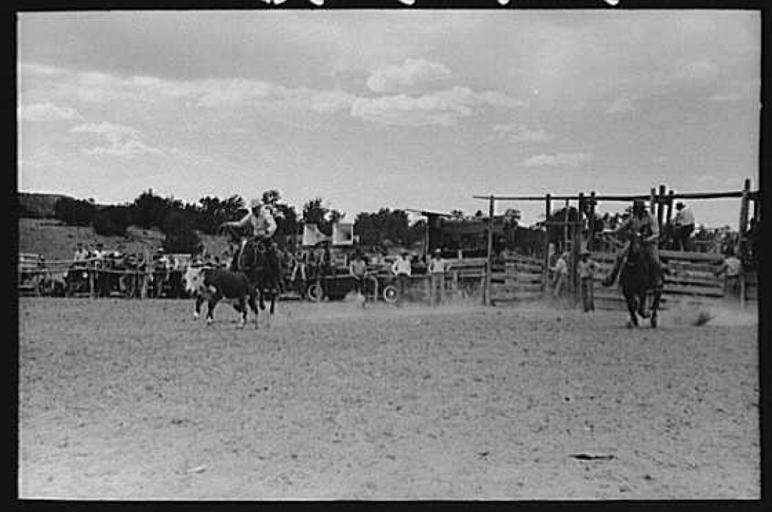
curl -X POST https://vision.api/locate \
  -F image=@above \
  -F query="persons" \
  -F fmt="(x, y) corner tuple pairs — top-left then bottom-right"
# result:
(553, 239), (602, 320)
(426, 246), (453, 308)
(710, 218), (757, 301)
(223, 197), (277, 241)
(18, 244), (238, 299)
(671, 202), (695, 255)
(604, 198), (678, 285)
(280, 239), (423, 303)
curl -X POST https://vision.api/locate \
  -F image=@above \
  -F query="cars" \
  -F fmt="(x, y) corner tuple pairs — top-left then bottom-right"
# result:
(304, 263), (399, 304)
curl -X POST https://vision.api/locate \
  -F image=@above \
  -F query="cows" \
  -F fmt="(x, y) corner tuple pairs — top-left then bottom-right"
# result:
(181, 262), (260, 331)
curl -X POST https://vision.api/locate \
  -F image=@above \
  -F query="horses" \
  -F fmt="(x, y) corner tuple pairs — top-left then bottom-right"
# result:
(619, 223), (663, 330)
(228, 234), (279, 317)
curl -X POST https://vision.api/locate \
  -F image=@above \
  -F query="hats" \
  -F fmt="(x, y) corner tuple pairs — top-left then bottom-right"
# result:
(249, 198), (263, 207)
(629, 200), (649, 207)
